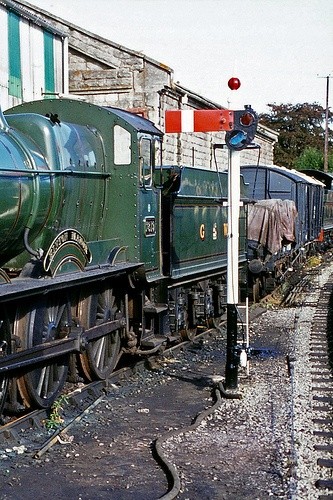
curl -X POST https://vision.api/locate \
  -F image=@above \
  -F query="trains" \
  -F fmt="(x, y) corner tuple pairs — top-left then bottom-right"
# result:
(0, 96), (333, 407)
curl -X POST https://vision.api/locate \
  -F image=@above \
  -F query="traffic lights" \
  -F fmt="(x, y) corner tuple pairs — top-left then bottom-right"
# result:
(224, 110), (256, 148)
